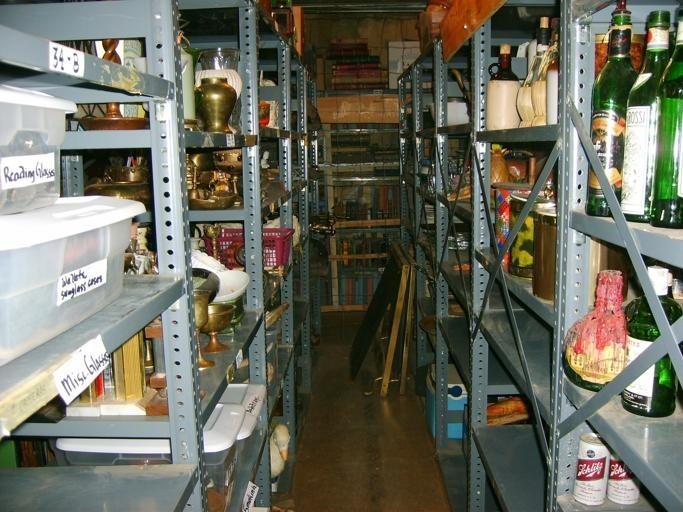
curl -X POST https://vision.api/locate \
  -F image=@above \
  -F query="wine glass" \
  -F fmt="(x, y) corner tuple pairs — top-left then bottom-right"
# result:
(193, 289), (234, 370)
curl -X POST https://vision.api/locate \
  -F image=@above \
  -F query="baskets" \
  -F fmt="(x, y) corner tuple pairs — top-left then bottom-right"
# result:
(200, 228), (295, 270)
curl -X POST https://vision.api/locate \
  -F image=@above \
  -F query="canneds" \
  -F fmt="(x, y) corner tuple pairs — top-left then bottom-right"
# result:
(607, 450), (640, 506)
(573, 433), (612, 507)
(492, 180), (558, 304)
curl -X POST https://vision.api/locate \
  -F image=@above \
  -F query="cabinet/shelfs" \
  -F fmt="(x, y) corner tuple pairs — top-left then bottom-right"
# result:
(0, 4), (322, 511)
(316, 99), (404, 314)
(393, 2), (683, 512)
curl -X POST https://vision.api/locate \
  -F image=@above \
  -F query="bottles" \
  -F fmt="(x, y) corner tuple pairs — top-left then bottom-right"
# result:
(582, 1), (683, 230)
(487, 16), (561, 128)
(560, 255), (683, 418)
(195, 78), (237, 132)
